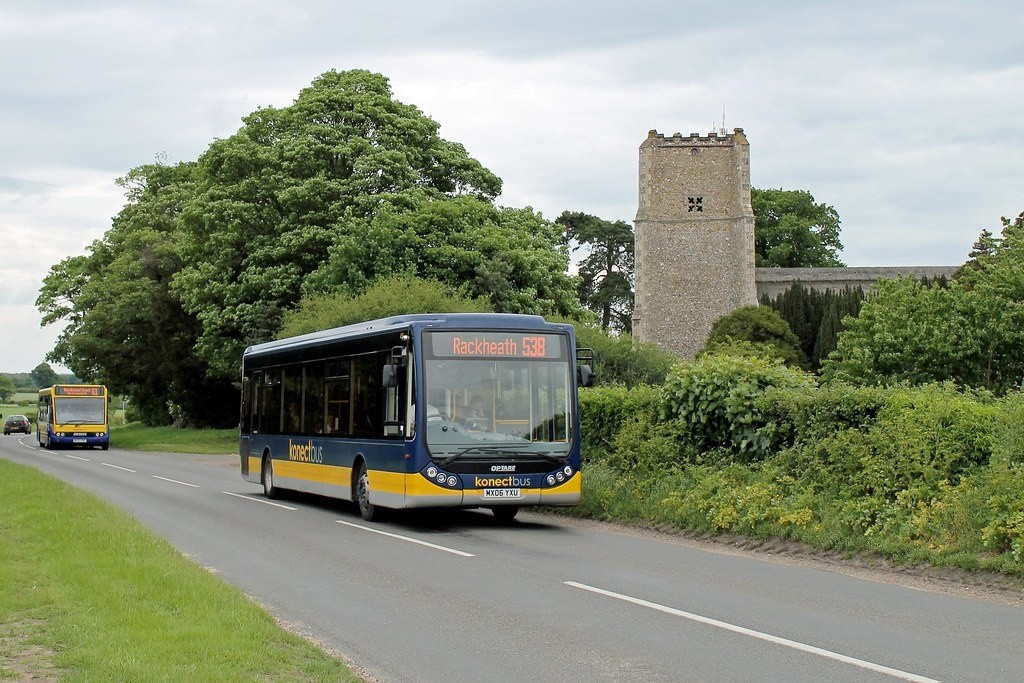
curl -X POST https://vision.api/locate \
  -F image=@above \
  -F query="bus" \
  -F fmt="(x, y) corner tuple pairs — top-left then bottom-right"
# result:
(35, 383), (112, 451)
(237, 311), (596, 527)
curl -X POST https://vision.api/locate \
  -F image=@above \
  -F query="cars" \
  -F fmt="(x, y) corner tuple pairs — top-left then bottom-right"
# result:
(3, 414), (33, 436)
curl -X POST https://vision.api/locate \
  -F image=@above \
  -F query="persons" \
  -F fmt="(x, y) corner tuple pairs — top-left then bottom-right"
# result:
(409, 402), (440, 430)
(286, 375), (336, 434)
(461, 394), (488, 431)
